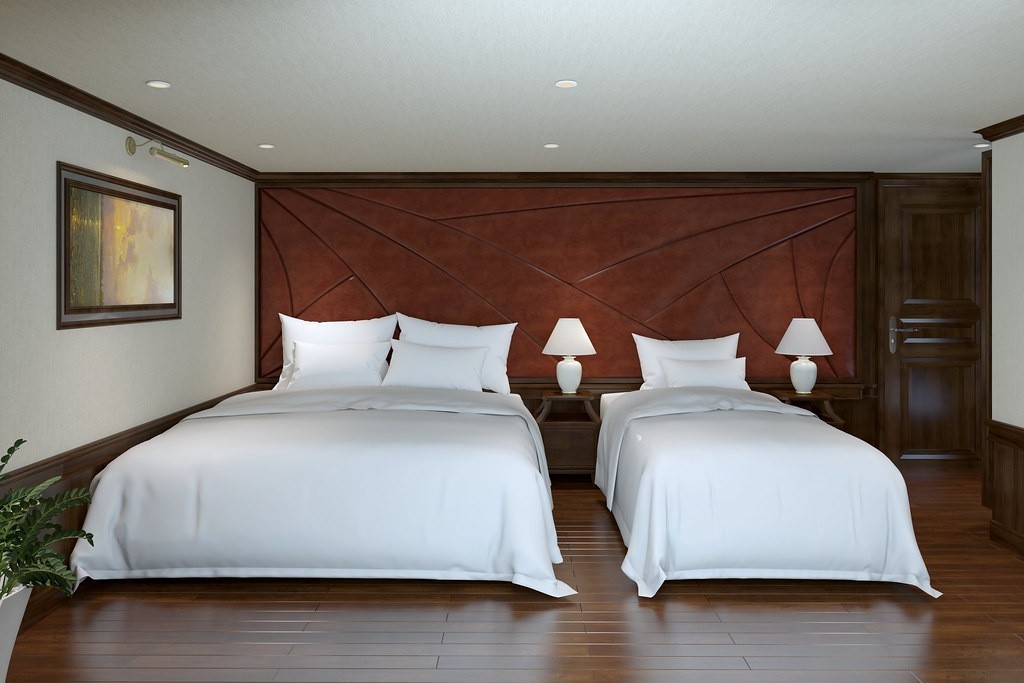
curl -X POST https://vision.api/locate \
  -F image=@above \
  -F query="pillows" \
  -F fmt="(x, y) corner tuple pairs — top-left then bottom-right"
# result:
(286, 341), (392, 389)
(381, 338), (490, 392)
(273, 312), (397, 395)
(658, 356), (753, 391)
(396, 311), (521, 395)
(629, 331), (741, 391)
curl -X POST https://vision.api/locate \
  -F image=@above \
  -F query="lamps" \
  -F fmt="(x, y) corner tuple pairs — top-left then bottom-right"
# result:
(125, 134), (190, 168)
(774, 318), (833, 393)
(542, 318), (597, 394)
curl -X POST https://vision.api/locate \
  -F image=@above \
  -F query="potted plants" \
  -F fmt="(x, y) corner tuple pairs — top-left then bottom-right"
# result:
(0, 439), (94, 683)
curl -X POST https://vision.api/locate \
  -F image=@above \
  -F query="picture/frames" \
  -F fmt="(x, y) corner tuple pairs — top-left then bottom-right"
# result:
(55, 160), (183, 328)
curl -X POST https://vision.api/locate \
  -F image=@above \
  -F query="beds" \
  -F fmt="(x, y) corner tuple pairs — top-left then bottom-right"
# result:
(68, 389), (578, 601)
(593, 387), (943, 601)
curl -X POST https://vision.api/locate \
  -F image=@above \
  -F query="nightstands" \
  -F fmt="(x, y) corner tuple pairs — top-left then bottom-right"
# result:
(774, 388), (847, 430)
(532, 390), (603, 487)
